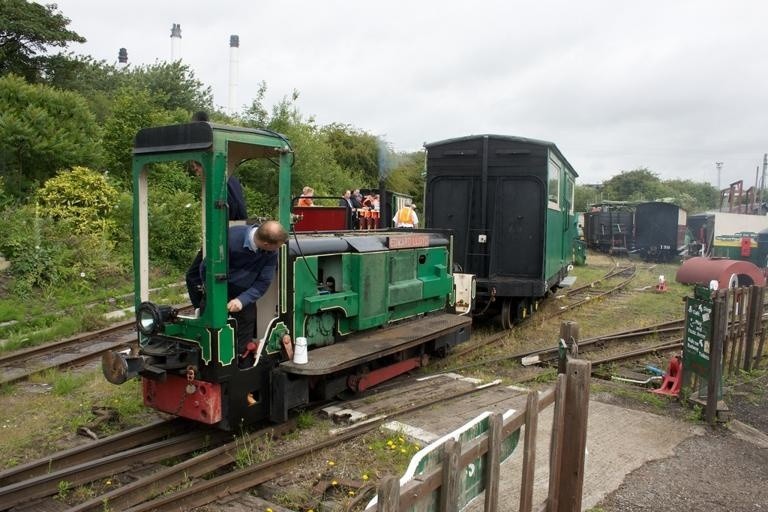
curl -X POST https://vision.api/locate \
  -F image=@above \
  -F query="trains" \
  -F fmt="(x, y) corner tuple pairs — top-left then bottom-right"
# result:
(582, 202), (688, 260)
(104, 120), (587, 429)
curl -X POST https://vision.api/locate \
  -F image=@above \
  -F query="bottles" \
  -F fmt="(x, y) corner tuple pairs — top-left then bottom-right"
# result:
(291, 337), (310, 366)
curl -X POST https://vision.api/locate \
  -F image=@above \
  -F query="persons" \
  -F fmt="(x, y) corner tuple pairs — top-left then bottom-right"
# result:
(390, 203), (419, 229)
(338, 188), (381, 230)
(296, 185), (324, 208)
(325, 276), (336, 293)
(186, 218), (290, 407)
